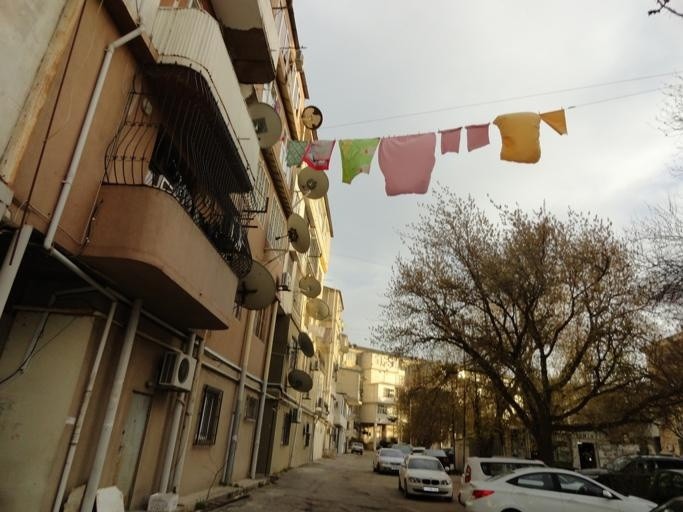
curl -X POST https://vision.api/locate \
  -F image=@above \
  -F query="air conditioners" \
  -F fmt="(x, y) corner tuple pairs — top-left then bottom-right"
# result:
(156, 350), (198, 392)
(289, 407), (302, 423)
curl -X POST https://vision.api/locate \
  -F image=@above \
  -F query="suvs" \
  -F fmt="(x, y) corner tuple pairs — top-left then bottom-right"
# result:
(574, 453), (683, 501)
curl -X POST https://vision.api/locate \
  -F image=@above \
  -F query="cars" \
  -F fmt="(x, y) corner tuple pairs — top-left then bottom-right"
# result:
(351, 442), (364, 455)
(398, 453), (454, 501)
(464, 465), (659, 512)
(372, 447), (405, 474)
